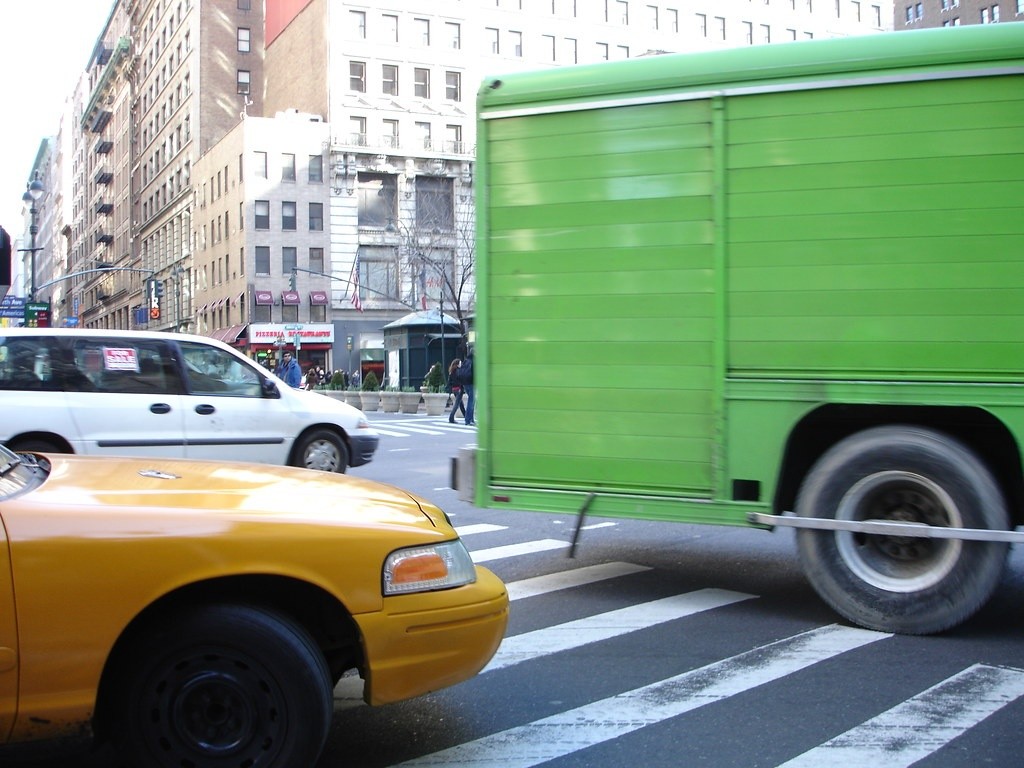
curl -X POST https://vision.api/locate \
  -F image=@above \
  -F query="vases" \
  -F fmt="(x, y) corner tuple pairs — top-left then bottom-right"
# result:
(450, 394), (468, 417)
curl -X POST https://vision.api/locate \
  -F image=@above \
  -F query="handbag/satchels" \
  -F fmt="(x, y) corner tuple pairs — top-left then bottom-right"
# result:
(447, 396), (453, 407)
(456, 360), (473, 381)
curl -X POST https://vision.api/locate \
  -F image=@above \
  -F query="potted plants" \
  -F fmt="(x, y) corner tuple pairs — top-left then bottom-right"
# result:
(419, 381), (429, 393)
(310, 385), (327, 396)
(397, 386), (422, 414)
(379, 386), (402, 412)
(325, 372), (345, 402)
(421, 361), (450, 416)
(344, 383), (362, 410)
(359, 370), (383, 411)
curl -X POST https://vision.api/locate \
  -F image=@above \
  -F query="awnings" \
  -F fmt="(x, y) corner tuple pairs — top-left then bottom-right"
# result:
(211, 324), (247, 342)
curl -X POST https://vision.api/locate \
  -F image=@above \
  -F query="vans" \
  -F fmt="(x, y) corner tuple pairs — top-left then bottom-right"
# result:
(0, 323), (381, 476)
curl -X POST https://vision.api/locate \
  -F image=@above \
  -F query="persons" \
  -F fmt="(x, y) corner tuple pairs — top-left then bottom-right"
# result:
(461, 348), (476, 426)
(448, 359), (466, 423)
(279, 350), (302, 388)
(306, 365), (360, 390)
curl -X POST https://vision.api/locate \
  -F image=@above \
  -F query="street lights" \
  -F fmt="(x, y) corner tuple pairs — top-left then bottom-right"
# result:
(21, 169), (44, 304)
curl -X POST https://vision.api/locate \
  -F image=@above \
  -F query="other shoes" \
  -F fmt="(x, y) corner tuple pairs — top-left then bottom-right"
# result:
(449, 421), (458, 424)
(465, 422), (474, 426)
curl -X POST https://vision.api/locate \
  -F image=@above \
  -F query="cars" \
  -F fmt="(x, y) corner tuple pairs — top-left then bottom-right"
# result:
(0, 436), (513, 766)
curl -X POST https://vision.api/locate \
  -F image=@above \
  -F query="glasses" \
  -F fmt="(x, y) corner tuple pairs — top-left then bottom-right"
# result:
(284, 354), (291, 357)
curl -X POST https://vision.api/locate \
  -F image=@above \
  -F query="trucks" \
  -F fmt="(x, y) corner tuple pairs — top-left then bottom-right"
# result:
(444, 19), (1024, 636)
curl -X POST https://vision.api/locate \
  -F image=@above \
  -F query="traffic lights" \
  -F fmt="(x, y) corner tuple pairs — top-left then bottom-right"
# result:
(289, 274), (296, 291)
(155, 279), (163, 298)
